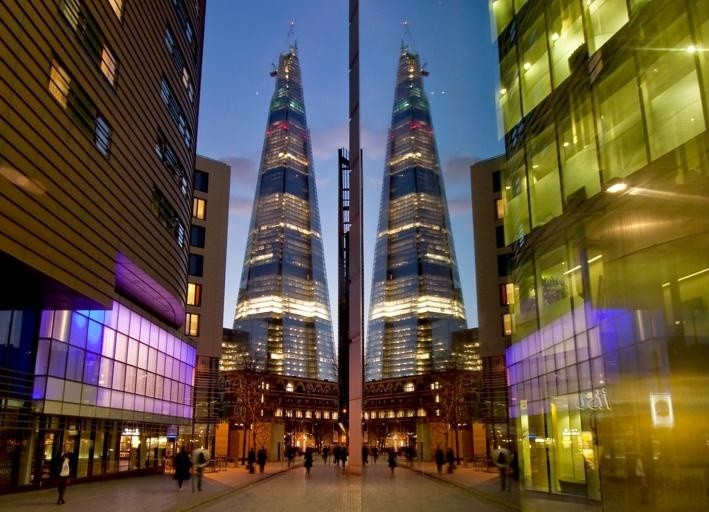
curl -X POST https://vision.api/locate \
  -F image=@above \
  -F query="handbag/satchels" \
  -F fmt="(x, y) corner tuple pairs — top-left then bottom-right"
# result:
(199, 449), (207, 464)
(247, 465), (249, 469)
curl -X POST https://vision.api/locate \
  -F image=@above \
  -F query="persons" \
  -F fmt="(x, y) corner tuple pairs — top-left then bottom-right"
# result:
(247, 448), (256, 474)
(434, 446), (445, 474)
(256, 446), (267, 474)
(53, 443), (75, 505)
(493, 446), (512, 492)
(284, 445), (417, 478)
(509, 448), (518, 484)
(172, 445), (193, 491)
(446, 448), (455, 474)
(191, 442), (210, 493)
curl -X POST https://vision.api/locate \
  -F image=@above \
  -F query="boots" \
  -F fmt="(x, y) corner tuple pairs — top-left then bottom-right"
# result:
(58, 490), (65, 504)
